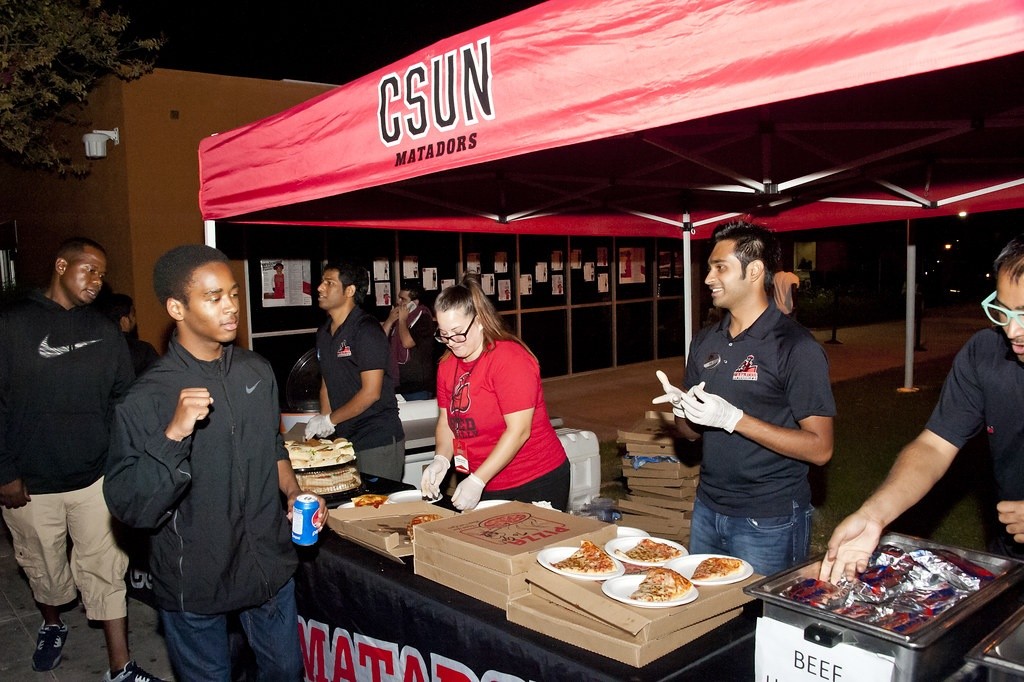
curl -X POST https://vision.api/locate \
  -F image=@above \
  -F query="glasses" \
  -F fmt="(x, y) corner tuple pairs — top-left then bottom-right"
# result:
(433, 313), (477, 343)
(981, 290), (1024, 329)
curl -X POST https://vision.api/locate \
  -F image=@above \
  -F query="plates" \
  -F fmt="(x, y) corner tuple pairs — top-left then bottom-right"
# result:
(605, 537), (689, 570)
(661, 553), (755, 585)
(601, 575), (702, 608)
(337, 500), (393, 509)
(536, 545), (626, 581)
(387, 490), (443, 504)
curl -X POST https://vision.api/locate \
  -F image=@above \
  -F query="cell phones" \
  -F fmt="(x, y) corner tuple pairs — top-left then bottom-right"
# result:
(407, 301), (416, 313)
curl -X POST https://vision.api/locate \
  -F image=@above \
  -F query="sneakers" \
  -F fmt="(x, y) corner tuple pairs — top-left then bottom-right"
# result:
(31, 619), (70, 671)
(104, 658), (168, 682)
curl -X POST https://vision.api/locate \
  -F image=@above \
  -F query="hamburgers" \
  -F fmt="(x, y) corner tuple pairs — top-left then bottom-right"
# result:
(282, 438), (355, 469)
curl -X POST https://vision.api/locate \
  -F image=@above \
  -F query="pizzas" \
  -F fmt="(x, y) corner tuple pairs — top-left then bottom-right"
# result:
(691, 558), (742, 580)
(615, 538), (682, 562)
(550, 540), (617, 573)
(632, 567), (692, 602)
(352, 494), (388, 508)
(407, 514), (442, 542)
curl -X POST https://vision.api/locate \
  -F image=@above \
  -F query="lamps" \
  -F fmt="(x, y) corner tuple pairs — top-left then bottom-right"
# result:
(81, 126), (120, 159)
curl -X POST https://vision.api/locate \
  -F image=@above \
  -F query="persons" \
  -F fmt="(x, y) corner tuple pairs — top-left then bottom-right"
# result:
(771, 256), (800, 315)
(103, 244), (329, 682)
(383, 281), (440, 401)
(420, 275), (570, 512)
(313, 259), (406, 481)
(0, 237), (162, 682)
(819, 236), (1024, 586)
(652, 220), (835, 576)
(104, 293), (160, 370)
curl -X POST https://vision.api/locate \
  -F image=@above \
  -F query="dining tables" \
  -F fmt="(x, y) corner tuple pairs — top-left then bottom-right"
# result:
(111, 472), (419, 610)
(661, 629), (756, 682)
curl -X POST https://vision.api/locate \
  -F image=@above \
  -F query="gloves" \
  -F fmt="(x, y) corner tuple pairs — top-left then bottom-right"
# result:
(314, 427), (336, 439)
(678, 386), (747, 435)
(450, 473), (487, 512)
(649, 370), (708, 419)
(421, 455), (453, 500)
(304, 413), (338, 440)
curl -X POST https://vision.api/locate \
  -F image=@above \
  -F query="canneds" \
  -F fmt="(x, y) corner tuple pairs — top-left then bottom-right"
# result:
(292, 494), (319, 546)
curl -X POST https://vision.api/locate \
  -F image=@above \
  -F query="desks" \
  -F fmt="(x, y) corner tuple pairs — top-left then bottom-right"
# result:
(296, 470), (757, 682)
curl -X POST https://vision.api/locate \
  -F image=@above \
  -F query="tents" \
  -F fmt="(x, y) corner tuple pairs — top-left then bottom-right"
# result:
(198, 0), (1024, 386)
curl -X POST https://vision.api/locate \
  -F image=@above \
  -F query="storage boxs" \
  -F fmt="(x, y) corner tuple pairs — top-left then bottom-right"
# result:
(325, 500), (462, 564)
(527, 563), (765, 639)
(414, 559), (527, 610)
(554, 427), (601, 514)
(615, 410), (700, 549)
(505, 593), (744, 668)
(743, 530), (1024, 682)
(963, 606), (1024, 682)
(413, 543), (528, 594)
(414, 500), (617, 576)
